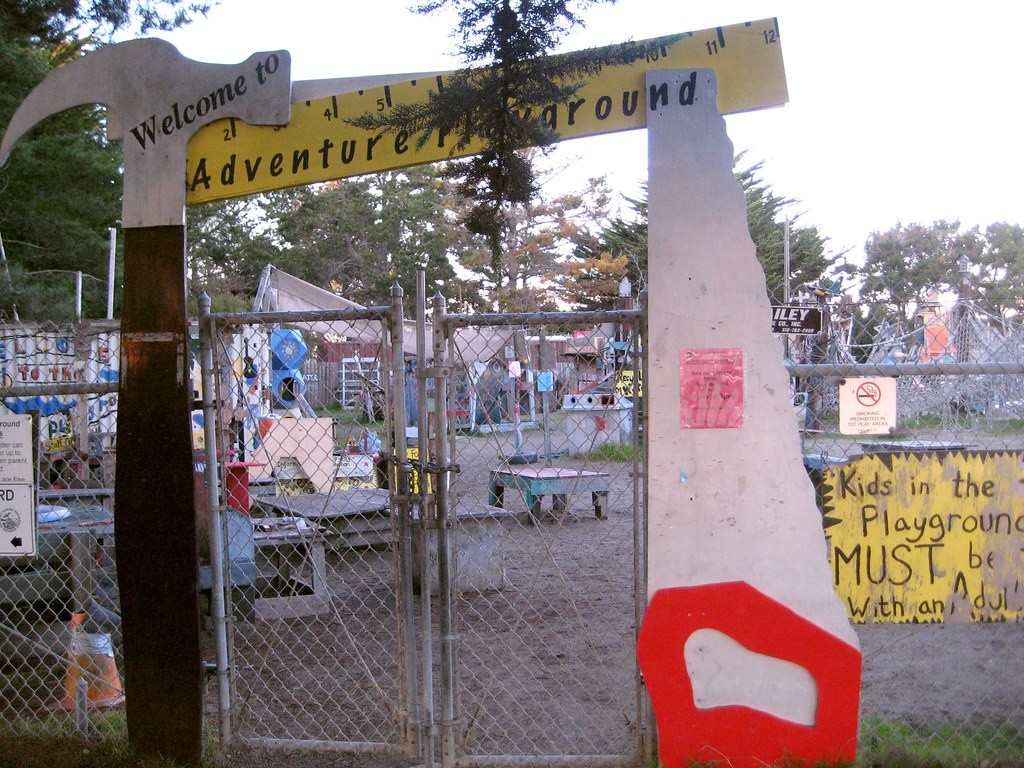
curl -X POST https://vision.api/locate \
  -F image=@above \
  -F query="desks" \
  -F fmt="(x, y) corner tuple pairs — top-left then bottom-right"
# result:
(249, 517), (334, 621)
(0, 506), (115, 689)
(859, 440), (977, 455)
(490, 469), (610, 525)
(384, 503), (525, 592)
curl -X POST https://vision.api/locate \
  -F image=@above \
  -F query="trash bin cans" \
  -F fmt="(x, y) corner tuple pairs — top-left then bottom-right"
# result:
(792, 391), (824, 435)
(562, 393), (634, 460)
(858, 439), (978, 452)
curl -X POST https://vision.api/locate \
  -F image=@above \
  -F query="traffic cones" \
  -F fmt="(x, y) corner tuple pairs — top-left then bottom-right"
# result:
(54, 592), (124, 709)
